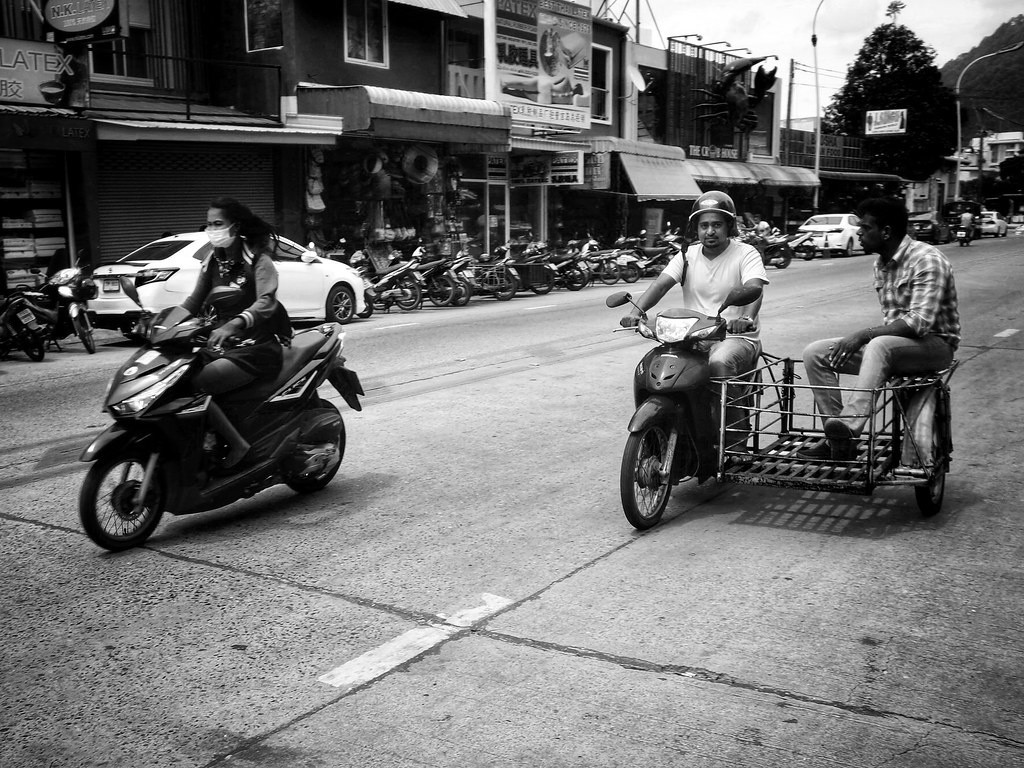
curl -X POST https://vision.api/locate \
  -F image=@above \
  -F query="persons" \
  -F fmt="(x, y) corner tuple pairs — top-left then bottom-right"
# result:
(179, 195), (293, 475)
(736, 214), (782, 240)
(961, 207), (975, 237)
(795, 196), (962, 458)
(868, 112), (872, 130)
(620, 190), (769, 463)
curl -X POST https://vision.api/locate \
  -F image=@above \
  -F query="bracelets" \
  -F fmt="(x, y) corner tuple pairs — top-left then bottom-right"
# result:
(739, 316), (753, 321)
(866, 328), (868, 337)
(869, 327), (873, 337)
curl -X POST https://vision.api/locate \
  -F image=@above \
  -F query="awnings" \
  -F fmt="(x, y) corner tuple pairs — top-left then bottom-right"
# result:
(595, 151), (705, 202)
(685, 156), (759, 185)
(811, 168), (902, 182)
(0, 103), (343, 145)
(392, 0), (471, 17)
(740, 161), (822, 186)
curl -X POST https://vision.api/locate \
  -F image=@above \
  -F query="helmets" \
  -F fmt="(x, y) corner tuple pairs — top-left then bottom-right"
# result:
(688, 190), (738, 237)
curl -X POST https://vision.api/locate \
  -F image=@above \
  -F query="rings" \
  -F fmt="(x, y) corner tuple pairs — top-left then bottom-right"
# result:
(841, 353), (846, 356)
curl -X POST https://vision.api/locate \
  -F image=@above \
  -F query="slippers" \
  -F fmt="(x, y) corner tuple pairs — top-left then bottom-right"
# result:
(727, 445), (752, 464)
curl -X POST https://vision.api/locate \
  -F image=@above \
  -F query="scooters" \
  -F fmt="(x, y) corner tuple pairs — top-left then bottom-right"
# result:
(954, 218), (978, 246)
(306, 218), (817, 319)
(0, 248), (95, 364)
(78, 274), (367, 550)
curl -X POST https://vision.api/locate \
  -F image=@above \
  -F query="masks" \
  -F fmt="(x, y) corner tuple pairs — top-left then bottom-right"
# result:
(205, 223), (236, 248)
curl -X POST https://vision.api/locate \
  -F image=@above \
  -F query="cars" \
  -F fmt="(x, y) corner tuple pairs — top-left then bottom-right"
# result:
(905, 210), (956, 245)
(793, 213), (873, 257)
(84, 230), (368, 346)
(942, 206), (1010, 247)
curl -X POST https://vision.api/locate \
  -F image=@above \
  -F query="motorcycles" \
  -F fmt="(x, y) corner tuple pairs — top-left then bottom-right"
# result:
(607, 190), (963, 531)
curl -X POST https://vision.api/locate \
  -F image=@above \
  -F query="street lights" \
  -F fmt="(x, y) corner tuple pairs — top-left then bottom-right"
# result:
(953, 40), (1024, 203)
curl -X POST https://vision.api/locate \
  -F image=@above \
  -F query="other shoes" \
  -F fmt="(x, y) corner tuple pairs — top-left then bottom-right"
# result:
(823, 417), (856, 462)
(797, 441), (830, 459)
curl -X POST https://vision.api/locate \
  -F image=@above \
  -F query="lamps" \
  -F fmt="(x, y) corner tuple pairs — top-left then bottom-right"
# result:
(764, 55), (778, 60)
(720, 48), (751, 53)
(700, 41), (731, 47)
(668, 34), (702, 40)
